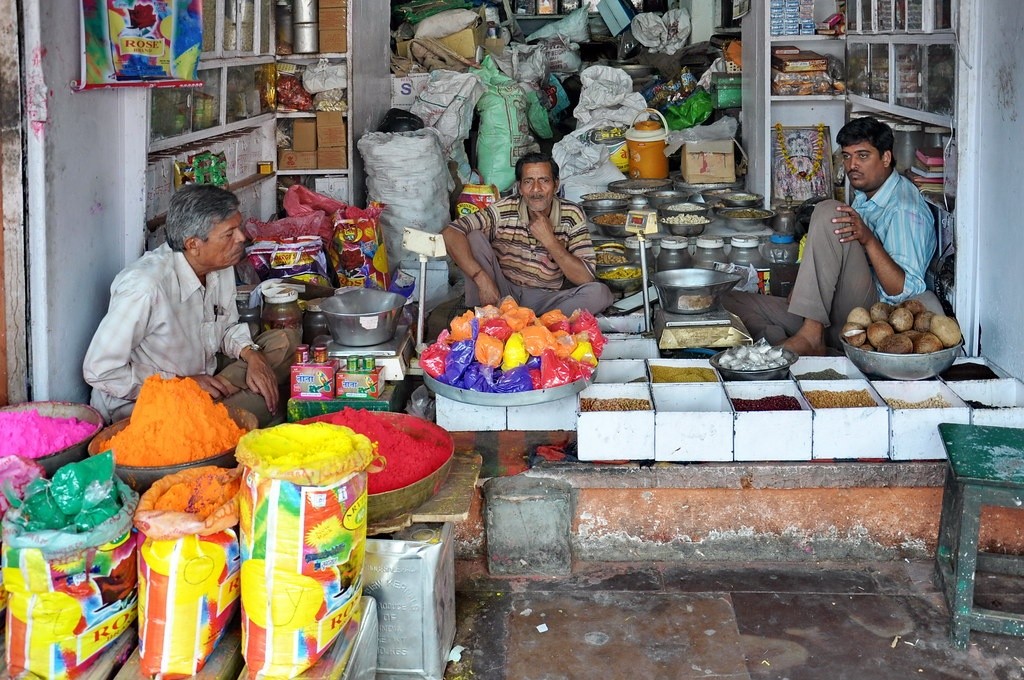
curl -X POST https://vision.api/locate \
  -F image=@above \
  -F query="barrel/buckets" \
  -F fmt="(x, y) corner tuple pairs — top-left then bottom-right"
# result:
(625, 108), (670, 179)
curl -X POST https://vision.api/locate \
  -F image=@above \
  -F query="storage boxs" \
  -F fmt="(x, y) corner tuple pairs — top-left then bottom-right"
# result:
(147, 0), (1024, 462)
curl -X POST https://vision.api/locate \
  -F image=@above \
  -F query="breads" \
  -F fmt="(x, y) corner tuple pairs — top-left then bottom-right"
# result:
(842, 299), (962, 355)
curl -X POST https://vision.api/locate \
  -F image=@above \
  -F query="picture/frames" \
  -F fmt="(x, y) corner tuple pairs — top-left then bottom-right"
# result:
(771, 126), (836, 207)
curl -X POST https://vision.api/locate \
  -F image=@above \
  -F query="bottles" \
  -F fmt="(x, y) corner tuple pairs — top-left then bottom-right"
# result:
(625, 204), (807, 296)
(275, 0), (292, 55)
(235, 288), (330, 348)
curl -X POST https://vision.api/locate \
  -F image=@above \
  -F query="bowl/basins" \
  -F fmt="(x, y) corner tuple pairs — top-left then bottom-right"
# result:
(577, 170), (777, 237)
(0, 401), (104, 479)
(614, 65), (654, 78)
(648, 269), (745, 315)
(288, 409), (454, 525)
(710, 347), (799, 382)
(319, 289), (407, 347)
(593, 242), (648, 293)
(88, 407), (259, 496)
(838, 333), (963, 381)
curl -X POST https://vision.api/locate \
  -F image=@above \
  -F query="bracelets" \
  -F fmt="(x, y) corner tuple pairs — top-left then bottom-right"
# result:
(472, 268), (483, 281)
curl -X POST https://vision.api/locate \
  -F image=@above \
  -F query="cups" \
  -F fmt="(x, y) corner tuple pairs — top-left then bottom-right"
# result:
(293, 23), (319, 54)
(292, 0), (320, 23)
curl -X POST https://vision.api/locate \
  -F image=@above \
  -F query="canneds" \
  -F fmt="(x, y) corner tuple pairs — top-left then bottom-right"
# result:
(346, 355), (375, 371)
(296, 344), (327, 363)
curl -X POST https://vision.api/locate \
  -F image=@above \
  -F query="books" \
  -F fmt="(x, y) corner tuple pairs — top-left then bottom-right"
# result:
(904, 147), (943, 194)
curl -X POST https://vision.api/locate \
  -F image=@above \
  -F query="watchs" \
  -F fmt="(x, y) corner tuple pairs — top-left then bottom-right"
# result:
(242, 344), (263, 362)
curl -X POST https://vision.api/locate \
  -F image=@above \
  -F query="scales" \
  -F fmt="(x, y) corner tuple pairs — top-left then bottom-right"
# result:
(624, 208), (753, 355)
(327, 226), (449, 381)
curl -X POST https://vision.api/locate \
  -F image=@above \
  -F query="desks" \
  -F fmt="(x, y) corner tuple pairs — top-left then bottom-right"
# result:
(935, 422), (1024, 649)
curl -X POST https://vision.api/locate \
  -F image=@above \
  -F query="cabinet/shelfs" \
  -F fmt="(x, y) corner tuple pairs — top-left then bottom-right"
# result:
(125, 0), (277, 266)
(742, 0), (845, 200)
(845, 0), (963, 130)
(277, 0), (392, 208)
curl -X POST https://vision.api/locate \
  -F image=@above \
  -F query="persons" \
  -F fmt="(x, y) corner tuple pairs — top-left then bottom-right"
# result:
(439, 151), (614, 318)
(83, 184), (300, 428)
(722, 117), (937, 357)
